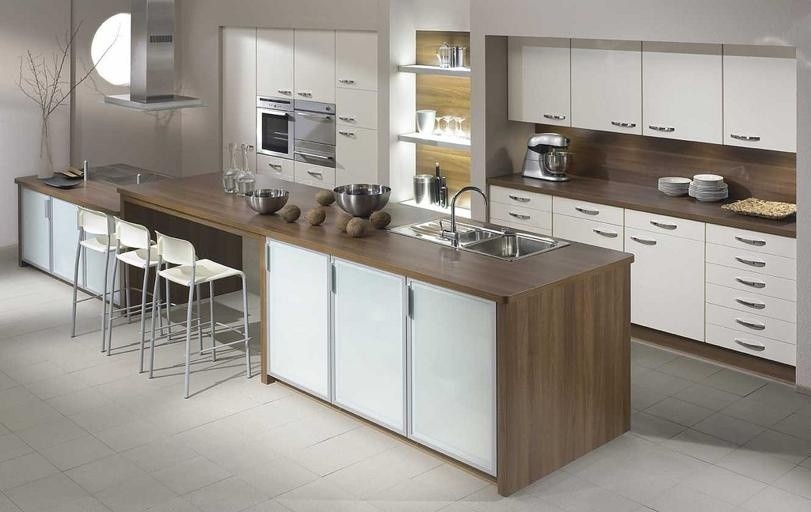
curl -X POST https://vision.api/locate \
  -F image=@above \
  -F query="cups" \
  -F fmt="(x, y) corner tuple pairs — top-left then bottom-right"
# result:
(416, 109), (436, 136)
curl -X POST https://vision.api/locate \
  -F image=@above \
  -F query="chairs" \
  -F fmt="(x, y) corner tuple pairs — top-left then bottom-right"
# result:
(69, 203), (255, 400)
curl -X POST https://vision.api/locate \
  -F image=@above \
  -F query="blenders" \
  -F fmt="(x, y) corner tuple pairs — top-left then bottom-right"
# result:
(520, 133), (573, 183)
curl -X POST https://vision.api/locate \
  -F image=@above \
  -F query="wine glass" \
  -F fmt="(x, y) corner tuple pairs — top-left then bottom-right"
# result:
(434, 115), (467, 138)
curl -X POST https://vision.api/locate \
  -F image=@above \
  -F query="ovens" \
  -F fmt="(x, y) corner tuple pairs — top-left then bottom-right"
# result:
(256, 95), (336, 170)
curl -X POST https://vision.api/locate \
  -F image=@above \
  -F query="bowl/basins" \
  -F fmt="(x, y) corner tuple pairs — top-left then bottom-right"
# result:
(333, 183), (392, 217)
(242, 189), (290, 214)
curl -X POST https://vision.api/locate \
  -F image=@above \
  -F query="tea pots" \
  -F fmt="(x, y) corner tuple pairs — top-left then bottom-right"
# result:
(433, 42), (452, 69)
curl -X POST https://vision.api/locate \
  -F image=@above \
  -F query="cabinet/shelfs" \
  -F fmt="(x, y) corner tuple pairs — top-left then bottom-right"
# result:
(15, 184), (53, 274)
(78, 202), (122, 308)
(257, 153), (337, 192)
(550, 198), (705, 348)
(328, 258), (409, 436)
(263, 238), (332, 410)
(256, 31), (335, 104)
(724, 44), (798, 148)
(51, 192), (85, 290)
(704, 218), (798, 371)
(507, 39), (572, 126)
(396, 58), (471, 221)
(336, 29), (380, 92)
(337, 87), (378, 188)
(486, 184), (550, 241)
(404, 261), (634, 475)
(571, 44), (723, 144)
(219, 27), (256, 180)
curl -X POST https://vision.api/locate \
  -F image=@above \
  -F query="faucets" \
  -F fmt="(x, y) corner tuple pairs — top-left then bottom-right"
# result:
(438, 186), (487, 246)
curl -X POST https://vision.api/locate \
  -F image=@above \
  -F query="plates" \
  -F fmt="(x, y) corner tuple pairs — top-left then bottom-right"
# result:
(657, 174), (729, 203)
(43, 177), (82, 188)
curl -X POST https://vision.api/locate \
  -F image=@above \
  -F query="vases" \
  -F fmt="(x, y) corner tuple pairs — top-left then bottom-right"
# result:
(33, 121), (55, 180)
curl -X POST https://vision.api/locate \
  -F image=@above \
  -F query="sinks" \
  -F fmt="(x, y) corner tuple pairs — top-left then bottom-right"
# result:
(452, 227), (497, 247)
(462, 232), (571, 262)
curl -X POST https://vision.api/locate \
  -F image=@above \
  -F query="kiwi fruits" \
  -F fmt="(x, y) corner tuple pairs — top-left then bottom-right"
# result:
(282, 188), (391, 237)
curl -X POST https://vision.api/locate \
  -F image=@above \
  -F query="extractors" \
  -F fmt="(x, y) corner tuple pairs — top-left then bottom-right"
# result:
(103, 1), (204, 113)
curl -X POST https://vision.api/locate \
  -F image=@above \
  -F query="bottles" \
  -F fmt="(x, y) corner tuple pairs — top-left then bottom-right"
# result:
(223, 140), (256, 197)
(451, 46), (468, 67)
(413, 162), (449, 210)
